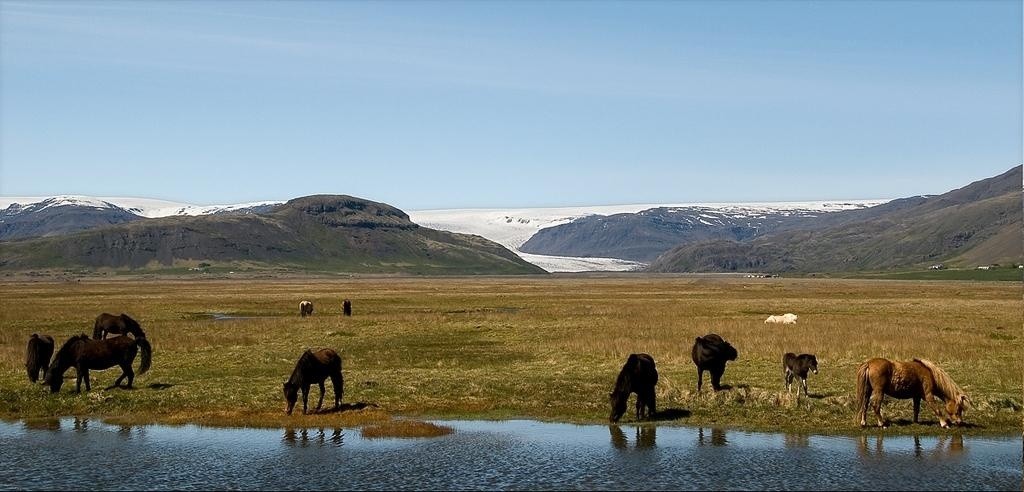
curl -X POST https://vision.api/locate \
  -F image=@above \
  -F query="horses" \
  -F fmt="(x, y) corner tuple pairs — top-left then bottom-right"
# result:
(857, 357), (975, 430)
(282, 347), (344, 416)
(691, 333), (737, 391)
(24, 311), (151, 394)
(343, 300), (351, 316)
(764, 312), (798, 325)
(608, 353), (658, 424)
(299, 300), (313, 317)
(782, 352), (818, 398)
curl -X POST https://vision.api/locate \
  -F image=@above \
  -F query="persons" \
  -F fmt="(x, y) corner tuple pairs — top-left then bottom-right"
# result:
(342, 298), (352, 316)
